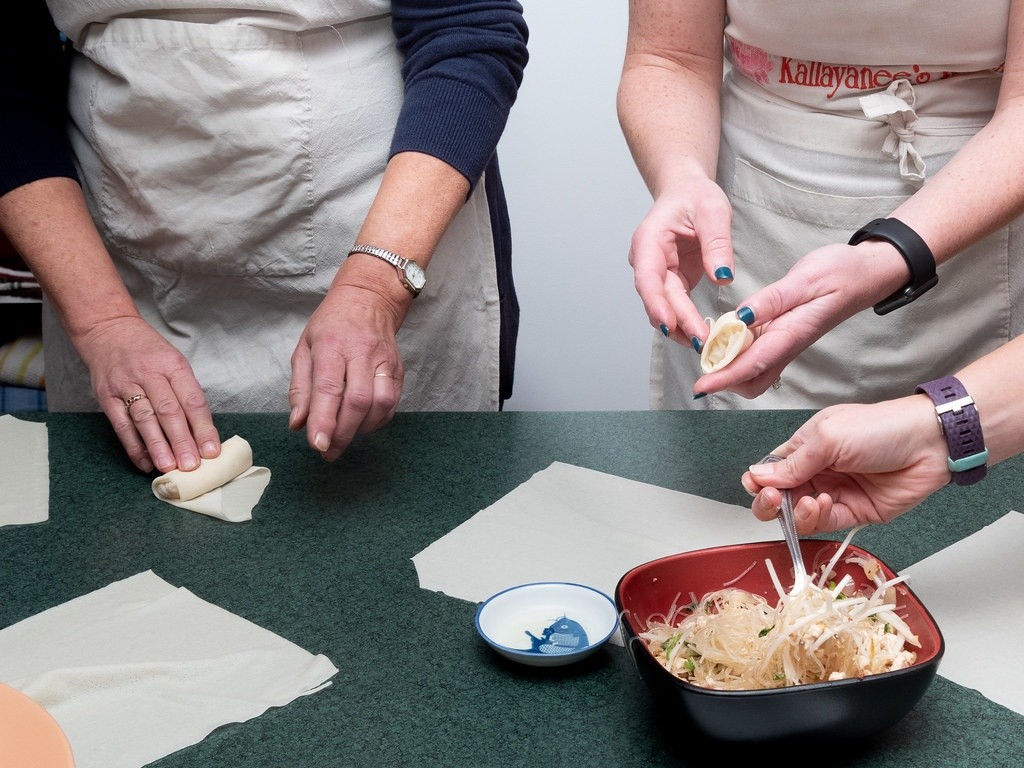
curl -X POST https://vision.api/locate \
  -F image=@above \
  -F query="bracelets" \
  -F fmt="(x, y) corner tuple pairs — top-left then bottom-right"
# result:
(914, 376), (989, 486)
(849, 218), (938, 316)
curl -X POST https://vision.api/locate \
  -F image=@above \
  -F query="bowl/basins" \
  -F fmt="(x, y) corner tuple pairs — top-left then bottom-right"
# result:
(614, 538), (945, 768)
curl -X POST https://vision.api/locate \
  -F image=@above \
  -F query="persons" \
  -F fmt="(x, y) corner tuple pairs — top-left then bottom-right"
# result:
(741, 334), (1024, 535)
(616, 0), (1024, 409)
(0, 0), (529, 472)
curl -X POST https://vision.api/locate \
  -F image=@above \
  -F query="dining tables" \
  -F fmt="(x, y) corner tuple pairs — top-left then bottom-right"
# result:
(0, 409), (1024, 768)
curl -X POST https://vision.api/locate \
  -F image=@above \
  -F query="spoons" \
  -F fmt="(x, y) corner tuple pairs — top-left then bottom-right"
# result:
(760, 455), (822, 598)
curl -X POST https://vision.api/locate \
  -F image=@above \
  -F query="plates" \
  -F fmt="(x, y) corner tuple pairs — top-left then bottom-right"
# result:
(475, 582), (620, 667)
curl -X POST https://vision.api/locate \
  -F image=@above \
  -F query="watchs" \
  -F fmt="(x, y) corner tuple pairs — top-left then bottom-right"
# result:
(348, 245), (426, 299)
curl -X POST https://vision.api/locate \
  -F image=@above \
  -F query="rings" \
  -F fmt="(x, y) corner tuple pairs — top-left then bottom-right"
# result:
(126, 394), (146, 410)
(772, 376), (781, 390)
(375, 374), (390, 378)
